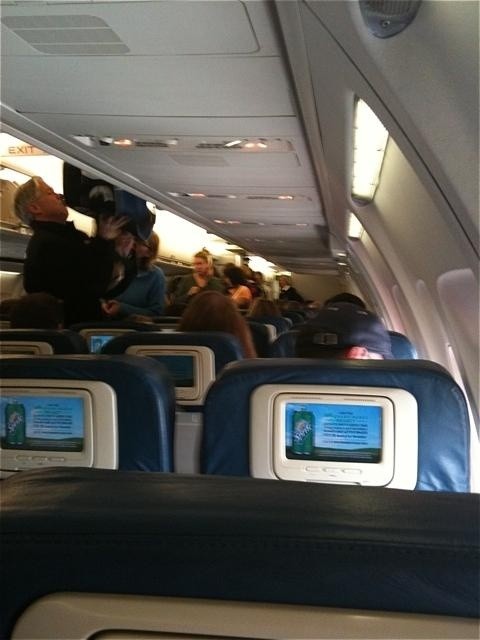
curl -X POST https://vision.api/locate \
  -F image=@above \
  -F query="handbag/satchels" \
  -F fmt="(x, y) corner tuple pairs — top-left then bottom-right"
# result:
(63, 162), (155, 239)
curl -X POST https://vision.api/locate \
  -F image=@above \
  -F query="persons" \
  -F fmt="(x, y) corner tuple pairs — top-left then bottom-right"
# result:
(9, 176), (394, 360)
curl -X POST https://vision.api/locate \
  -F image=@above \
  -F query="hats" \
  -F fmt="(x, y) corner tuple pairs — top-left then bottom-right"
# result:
(305, 301), (394, 359)
